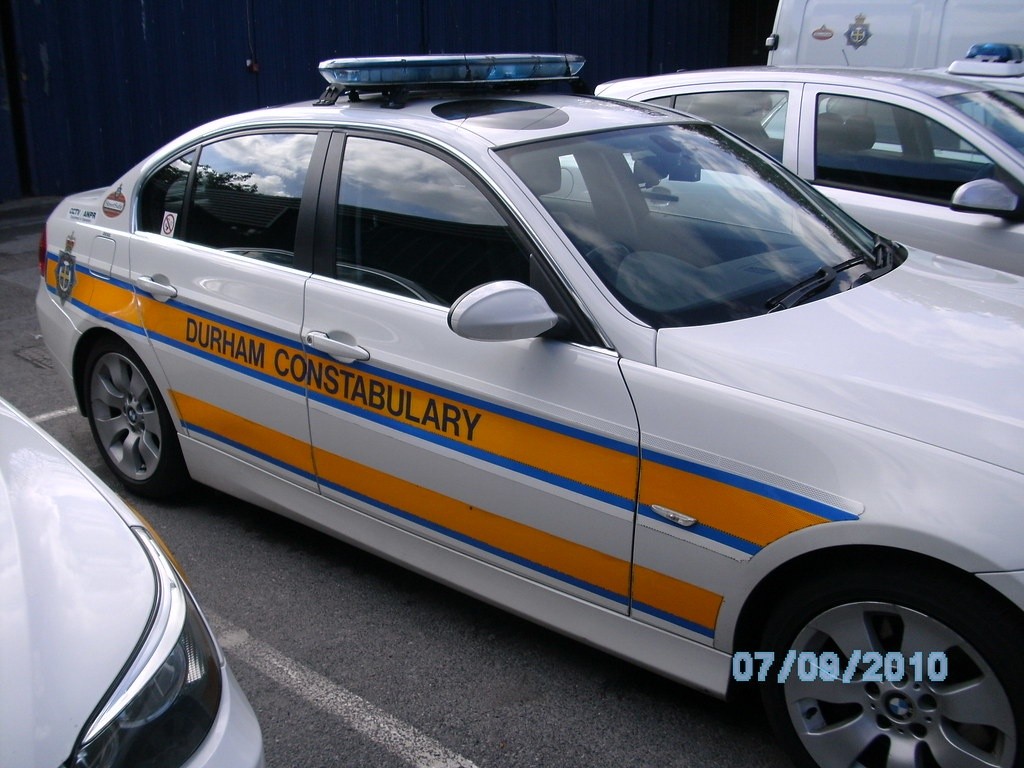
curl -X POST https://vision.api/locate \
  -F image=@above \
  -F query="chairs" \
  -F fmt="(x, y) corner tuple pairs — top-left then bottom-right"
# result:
(362, 154), (591, 302)
(817, 112), (887, 187)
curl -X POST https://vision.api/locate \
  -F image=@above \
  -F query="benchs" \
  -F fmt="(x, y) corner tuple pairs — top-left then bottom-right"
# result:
(705, 114), (784, 167)
(163, 167), (300, 248)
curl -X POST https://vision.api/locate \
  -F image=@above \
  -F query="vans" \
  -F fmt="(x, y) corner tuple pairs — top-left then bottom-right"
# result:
(766, 0), (1024, 99)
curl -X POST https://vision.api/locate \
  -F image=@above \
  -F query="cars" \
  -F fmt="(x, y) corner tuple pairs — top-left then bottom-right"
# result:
(1, 394), (264, 768)
(41, 43), (1024, 768)
(762, 41), (1024, 168)
(538, 67), (1024, 278)
